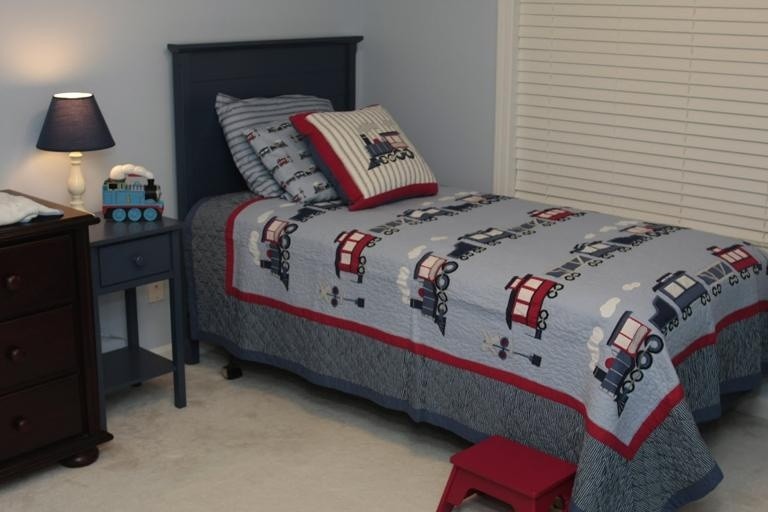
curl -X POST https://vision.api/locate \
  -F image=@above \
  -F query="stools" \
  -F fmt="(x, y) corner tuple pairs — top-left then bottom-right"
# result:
(434, 435), (577, 511)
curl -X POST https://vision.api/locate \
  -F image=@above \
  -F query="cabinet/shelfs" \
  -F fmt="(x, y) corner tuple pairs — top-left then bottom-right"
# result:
(0, 189), (101, 482)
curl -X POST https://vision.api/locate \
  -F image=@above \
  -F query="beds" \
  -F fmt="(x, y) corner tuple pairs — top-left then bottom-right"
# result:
(166, 35), (768, 511)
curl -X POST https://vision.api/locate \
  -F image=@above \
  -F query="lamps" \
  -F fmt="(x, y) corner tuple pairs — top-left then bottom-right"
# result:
(35, 91), (117, 217)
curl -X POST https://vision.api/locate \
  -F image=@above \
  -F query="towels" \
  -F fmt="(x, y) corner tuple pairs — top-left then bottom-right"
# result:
(0, 191), (65, 226)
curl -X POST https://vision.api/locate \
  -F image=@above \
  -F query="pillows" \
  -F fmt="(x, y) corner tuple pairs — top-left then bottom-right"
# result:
(214, 92), (437, 211)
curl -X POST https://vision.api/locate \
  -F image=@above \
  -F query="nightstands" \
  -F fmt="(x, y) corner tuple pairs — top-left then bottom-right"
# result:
(88, 210), (188, 430)
